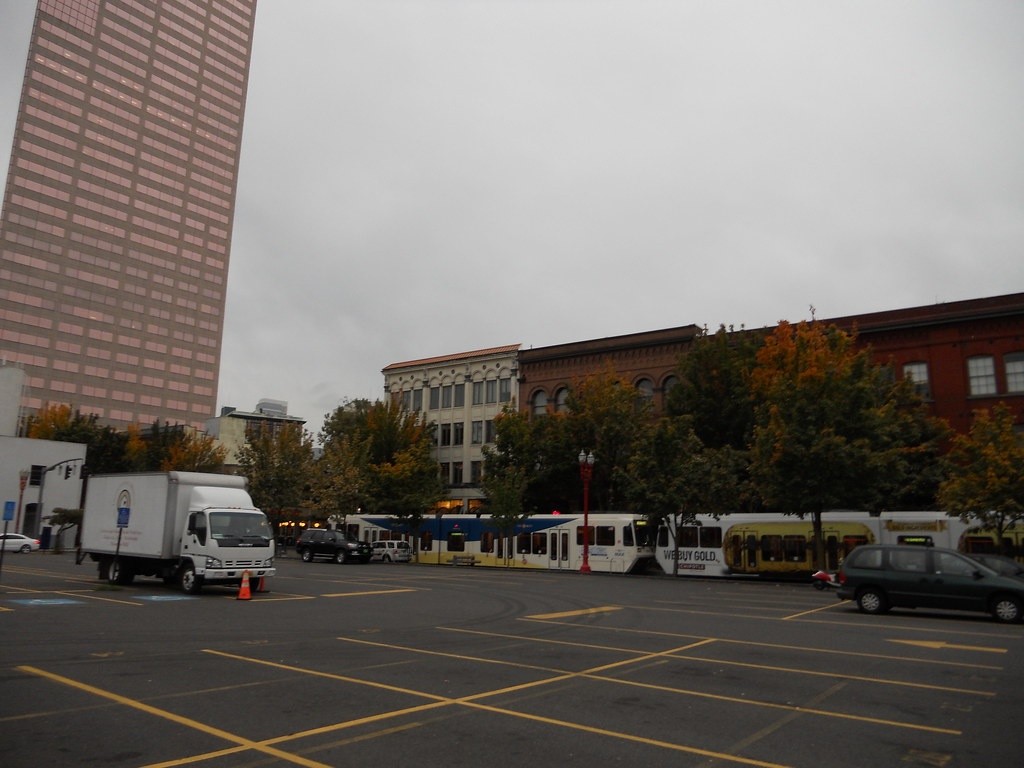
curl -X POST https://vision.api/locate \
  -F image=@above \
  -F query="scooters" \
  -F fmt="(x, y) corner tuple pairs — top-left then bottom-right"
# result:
(812, 570), (843, 591)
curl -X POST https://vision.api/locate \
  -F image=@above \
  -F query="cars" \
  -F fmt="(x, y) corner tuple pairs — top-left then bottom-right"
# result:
(935, 553), (1023, 581)
(836, 543), (1024, 625)
(0, 532), (40, 554)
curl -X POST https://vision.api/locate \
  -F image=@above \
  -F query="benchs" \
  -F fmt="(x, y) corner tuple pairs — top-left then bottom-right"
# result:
(446, 554), (481, 568)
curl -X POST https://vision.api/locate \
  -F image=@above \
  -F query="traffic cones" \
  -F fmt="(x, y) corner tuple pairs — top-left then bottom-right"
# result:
(256, 576), (270, 593)
(235, 570), (253, 600)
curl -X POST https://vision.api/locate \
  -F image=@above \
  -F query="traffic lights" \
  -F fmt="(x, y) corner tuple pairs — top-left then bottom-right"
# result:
(65, 465), (72, 479)
(79, 465), (88, 479)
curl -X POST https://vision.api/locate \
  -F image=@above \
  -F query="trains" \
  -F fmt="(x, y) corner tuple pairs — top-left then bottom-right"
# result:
(326, 510), (1023, 587)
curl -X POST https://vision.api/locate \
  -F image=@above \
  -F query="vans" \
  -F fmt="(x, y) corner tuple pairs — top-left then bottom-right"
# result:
(369, 540), (413, 564)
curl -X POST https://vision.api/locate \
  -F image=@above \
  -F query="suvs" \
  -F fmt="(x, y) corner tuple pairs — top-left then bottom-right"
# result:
(296, 527), (372, 564)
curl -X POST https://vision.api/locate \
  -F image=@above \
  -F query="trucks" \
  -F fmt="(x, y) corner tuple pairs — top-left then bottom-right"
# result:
(79, 470), (276, 595)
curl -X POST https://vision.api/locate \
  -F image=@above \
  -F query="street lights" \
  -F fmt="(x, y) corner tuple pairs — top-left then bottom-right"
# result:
(14, 469), (30, 534)
(578, 449), (595, 575)
